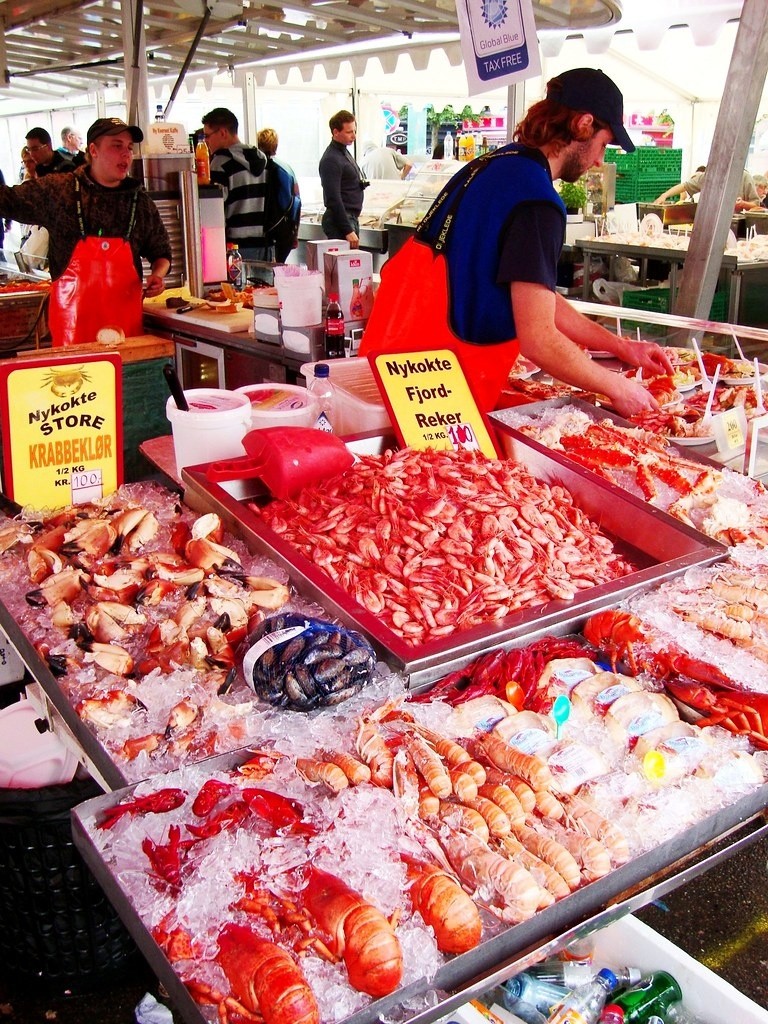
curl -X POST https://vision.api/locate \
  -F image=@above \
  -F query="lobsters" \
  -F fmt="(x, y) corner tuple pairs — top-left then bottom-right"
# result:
(96, 779), (484, 1024)
(406, 610), (768, 751)
(232, 689), (632, 926)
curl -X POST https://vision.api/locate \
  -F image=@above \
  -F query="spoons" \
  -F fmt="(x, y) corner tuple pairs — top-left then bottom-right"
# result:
(643, 749), (664, 782)
(553, 696), (570, 739)
(506, 682), (525, 712)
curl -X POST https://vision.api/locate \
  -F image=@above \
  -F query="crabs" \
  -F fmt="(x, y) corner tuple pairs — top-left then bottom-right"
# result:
(451, 657), (763, 852)
(0, 502), (291, 760)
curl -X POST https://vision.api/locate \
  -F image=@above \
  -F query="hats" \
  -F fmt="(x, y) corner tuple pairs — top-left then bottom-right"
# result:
(87, 118), (144, 144)
(547, 68), (636, 152)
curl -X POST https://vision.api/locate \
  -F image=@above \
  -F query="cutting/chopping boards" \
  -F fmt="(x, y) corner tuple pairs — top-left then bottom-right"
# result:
(143, 296), (254, 334)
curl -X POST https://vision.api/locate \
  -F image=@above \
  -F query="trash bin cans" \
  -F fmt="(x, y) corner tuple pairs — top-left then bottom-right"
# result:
(0, 781), (151, 1004)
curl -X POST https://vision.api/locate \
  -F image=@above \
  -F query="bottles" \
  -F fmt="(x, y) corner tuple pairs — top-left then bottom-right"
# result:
(305, 365), (335, 438)
(324, 293), (345, 359)
(444, 130), (490, 162)
(195, 135), (210, 186)
(445, 929), (716, 1024)
(583, 192), (593, 217)
(350, 279), (364, 318)
(155, 105), (164, 123)
(226, 241), (242, 287)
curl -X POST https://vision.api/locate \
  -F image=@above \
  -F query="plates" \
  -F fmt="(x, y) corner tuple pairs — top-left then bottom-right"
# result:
(509, 340), (768, 445)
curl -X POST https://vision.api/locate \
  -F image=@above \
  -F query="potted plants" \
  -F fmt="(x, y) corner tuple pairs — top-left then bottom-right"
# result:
(558, 181), (590, 215)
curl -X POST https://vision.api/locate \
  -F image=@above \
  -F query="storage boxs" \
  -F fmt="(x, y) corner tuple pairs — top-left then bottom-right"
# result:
(621, 287), (725, 340)
(603, 144), (679, 209)
(307, 239), (350, 273)
(122, 360), (173, 482)
(324, 249), (373, 323)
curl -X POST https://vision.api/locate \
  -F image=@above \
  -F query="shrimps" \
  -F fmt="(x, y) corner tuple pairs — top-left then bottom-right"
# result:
(671, 562), (768, 662)
(247, 442), (637, 647)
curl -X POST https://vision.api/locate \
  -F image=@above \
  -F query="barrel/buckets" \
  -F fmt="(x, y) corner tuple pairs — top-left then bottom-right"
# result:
(274, 274), (325, 327)
(166, 387), (251, 481)
(234, 383), (317, 431)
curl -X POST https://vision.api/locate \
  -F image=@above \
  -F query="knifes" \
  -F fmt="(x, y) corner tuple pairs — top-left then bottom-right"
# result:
(177, 303), (206, 314)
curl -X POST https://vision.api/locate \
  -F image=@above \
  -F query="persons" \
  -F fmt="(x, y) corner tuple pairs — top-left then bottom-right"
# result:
(361, 143), (412, 180)
(653, 167), (768, 213)
(19, 107), (301, 285)
(318, 110), (363, 249)
(0, 117), (172, 349)
(433, 144), (456, 160)
(355, 68), (674, 459)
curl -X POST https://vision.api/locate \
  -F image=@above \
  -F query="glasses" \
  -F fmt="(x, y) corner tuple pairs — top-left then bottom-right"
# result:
(26, 143), (50, 153)
(203, 127), (231, 138)
(21, 160), (34, 166)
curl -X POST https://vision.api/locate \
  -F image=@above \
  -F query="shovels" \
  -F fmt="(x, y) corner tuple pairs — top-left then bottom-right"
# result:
(206, 426), (355, 500)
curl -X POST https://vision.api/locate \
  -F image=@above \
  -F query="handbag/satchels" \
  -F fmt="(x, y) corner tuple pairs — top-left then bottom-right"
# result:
(265, 155), (301, 242)
